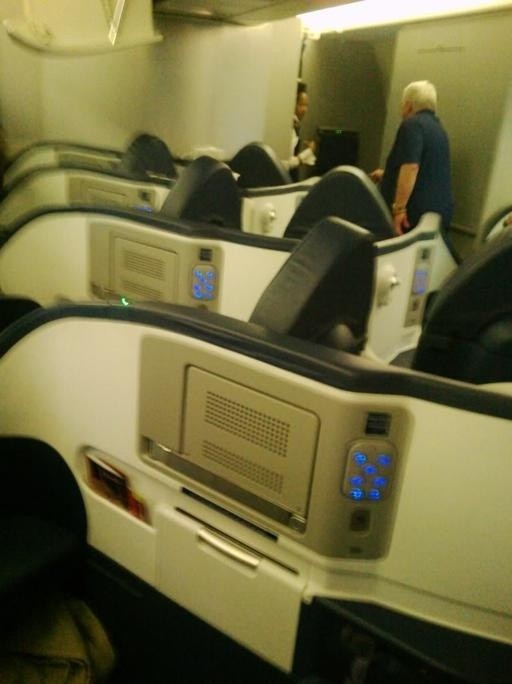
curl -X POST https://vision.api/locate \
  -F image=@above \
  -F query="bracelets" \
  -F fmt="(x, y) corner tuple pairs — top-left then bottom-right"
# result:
(391, 202), (407, 214)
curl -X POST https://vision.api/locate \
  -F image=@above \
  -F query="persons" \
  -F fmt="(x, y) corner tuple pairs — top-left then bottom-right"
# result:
(288, 80), (318, 181)
(371, 75), (455, 237)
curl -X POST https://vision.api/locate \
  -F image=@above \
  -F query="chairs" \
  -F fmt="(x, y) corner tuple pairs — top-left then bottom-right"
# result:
(114, 130), (512, 384)
